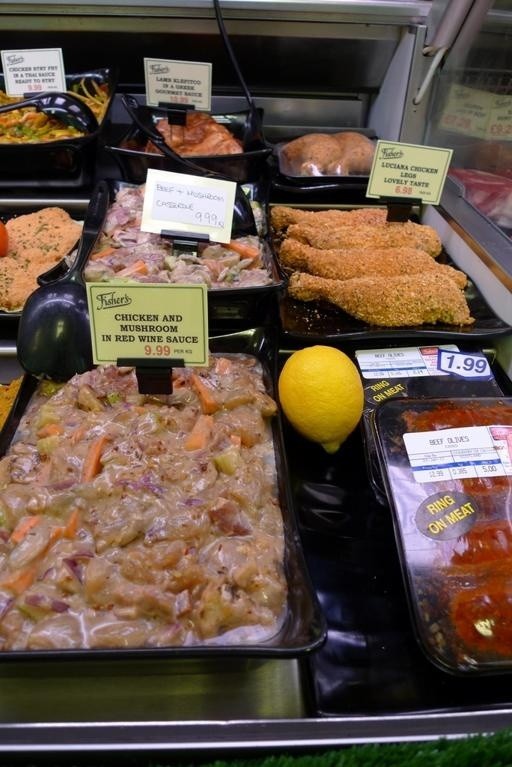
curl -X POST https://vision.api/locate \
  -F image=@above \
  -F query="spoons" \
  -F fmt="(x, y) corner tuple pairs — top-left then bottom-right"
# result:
(18, 178), (111, 382)
(120, 91), (256, 233)
(0, 90), (100, 133)
(213, 0), (261, 142)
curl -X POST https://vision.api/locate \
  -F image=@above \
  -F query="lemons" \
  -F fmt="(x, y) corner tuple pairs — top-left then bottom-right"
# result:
(278, 344), (364, 453)
